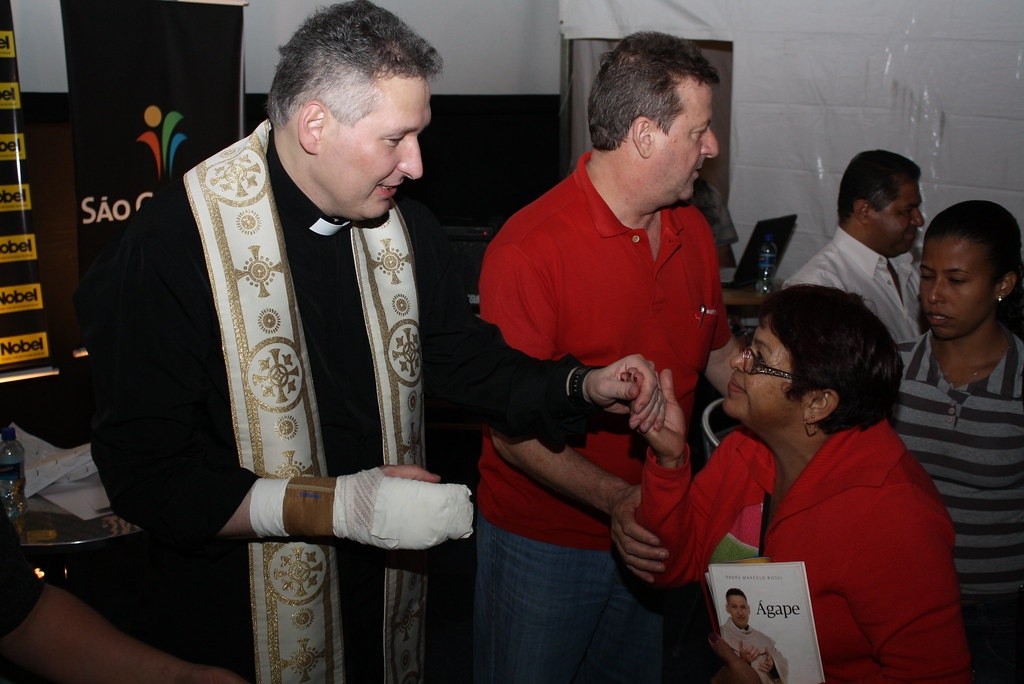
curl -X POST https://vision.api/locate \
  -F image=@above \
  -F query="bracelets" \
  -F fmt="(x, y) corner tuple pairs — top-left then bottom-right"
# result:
(568, 365), (605, 402)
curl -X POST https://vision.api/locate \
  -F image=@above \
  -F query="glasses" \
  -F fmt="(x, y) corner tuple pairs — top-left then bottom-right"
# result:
(742, 345), (794, 379)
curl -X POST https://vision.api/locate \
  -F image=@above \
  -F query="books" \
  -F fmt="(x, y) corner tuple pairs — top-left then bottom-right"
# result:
(704, 557), (825, 684)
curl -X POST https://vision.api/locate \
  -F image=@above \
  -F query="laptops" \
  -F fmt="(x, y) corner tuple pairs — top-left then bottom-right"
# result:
(716, 213), (797, 287)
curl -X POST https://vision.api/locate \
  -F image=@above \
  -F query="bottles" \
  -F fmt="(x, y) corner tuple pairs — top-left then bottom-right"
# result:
(755, 234), (777, 294)
(0, 427), (28, 518)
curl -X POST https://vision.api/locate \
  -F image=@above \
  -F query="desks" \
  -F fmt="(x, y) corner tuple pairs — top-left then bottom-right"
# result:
(721, 278), (781, 336)
(0, 460), (149, 597)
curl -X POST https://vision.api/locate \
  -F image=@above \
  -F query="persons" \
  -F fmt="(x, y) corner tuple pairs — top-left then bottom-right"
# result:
(468, 32), (745, 684)
(90, 0), (663, 684)
(623, 284), (970, 684)
(720, 588), (789, 684)
(0, 503), (247, 684)
(884, 199), (1024, 684)
(790, 150), (927, 339)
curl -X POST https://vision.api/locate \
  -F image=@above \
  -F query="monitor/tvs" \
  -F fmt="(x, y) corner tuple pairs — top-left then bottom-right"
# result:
(401, 92), (564, 239)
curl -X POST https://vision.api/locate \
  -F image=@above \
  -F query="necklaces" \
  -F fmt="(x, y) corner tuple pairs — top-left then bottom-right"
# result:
(967, 359), (997, 382)
